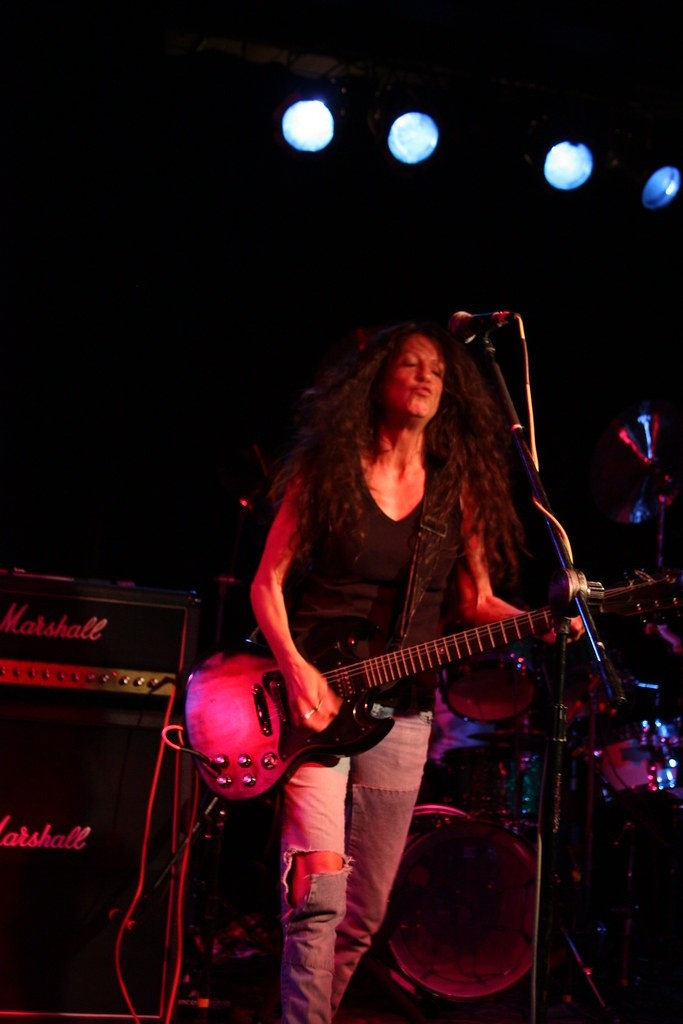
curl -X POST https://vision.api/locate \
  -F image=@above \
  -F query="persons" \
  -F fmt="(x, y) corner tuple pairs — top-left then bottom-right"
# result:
(250, 324), (586, 1023)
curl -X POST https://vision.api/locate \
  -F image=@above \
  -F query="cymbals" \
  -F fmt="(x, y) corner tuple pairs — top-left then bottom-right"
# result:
(466, 729), (548, 741)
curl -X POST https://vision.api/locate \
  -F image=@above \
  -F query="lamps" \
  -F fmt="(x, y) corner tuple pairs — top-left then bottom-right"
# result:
(364, 84), (443, 167)
(501, 99), (597, 192)
(603, 129), (682, 213)
(276, 73), (349, 156)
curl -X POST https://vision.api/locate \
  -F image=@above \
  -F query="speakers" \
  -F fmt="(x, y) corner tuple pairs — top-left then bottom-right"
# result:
(0, 700), (187, 1024)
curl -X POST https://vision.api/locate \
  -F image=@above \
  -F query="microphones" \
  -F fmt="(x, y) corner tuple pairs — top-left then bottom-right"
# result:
(107, 906), (139, 932)
(449, 310), (520, 343)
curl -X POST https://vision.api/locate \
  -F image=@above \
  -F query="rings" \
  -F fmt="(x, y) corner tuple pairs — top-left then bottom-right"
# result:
(314, 700), (322, 711)
(304, 710), (314, 719)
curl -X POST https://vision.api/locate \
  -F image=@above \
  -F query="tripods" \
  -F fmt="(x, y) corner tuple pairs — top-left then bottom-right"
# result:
(549, 666), (683, 1024)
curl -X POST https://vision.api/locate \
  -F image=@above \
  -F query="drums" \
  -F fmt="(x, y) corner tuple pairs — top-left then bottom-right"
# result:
(590, 710), (680, 792)
(377, 803), (540, 1000)
(434, 604), (540, 725)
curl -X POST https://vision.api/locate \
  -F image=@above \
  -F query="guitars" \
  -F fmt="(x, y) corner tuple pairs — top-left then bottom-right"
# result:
(177, 567), (683, 808)
